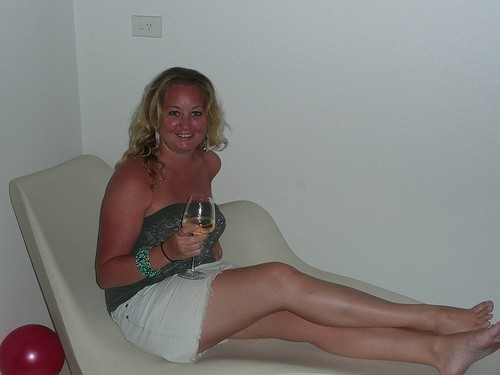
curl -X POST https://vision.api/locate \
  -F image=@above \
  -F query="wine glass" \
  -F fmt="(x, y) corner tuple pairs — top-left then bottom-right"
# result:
(177, 196), (215, 280)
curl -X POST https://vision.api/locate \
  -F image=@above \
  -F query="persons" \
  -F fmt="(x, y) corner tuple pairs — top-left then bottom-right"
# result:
(94, 67), (500, 375)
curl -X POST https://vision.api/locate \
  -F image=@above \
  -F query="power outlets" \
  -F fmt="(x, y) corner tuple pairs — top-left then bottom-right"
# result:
(132, 15), (162, 38)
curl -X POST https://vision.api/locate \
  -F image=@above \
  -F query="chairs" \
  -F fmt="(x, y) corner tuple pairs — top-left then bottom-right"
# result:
(9, 154), (500, 375)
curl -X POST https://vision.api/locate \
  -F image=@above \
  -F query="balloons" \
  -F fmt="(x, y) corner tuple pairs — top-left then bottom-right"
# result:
(0, 324), (66, 375)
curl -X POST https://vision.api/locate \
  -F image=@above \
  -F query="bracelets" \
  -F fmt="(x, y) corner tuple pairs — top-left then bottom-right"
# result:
(160, 242), (176, 264)
(135, 246), (161, 279)
